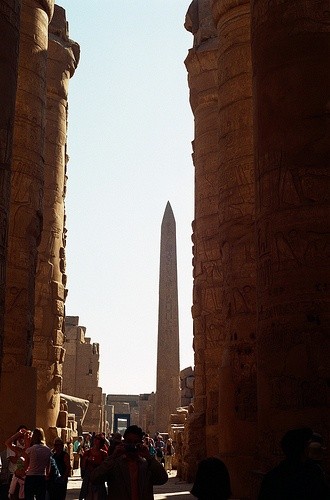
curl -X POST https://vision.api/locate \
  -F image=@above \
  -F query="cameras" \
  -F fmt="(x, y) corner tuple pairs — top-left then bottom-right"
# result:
(123, 439), (145, 450)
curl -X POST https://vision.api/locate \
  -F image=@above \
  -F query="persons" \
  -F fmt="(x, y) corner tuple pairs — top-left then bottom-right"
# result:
(142, 431), (175, 471)
(189, 456), (233, 500)
(80, 434), (123, 500)
(74, 430), (94, 458)
(5, 424), (71, 500)
(99, 431), (110, 451)
(257, 428), (330, 500)
(91, 425), (168, 500)
